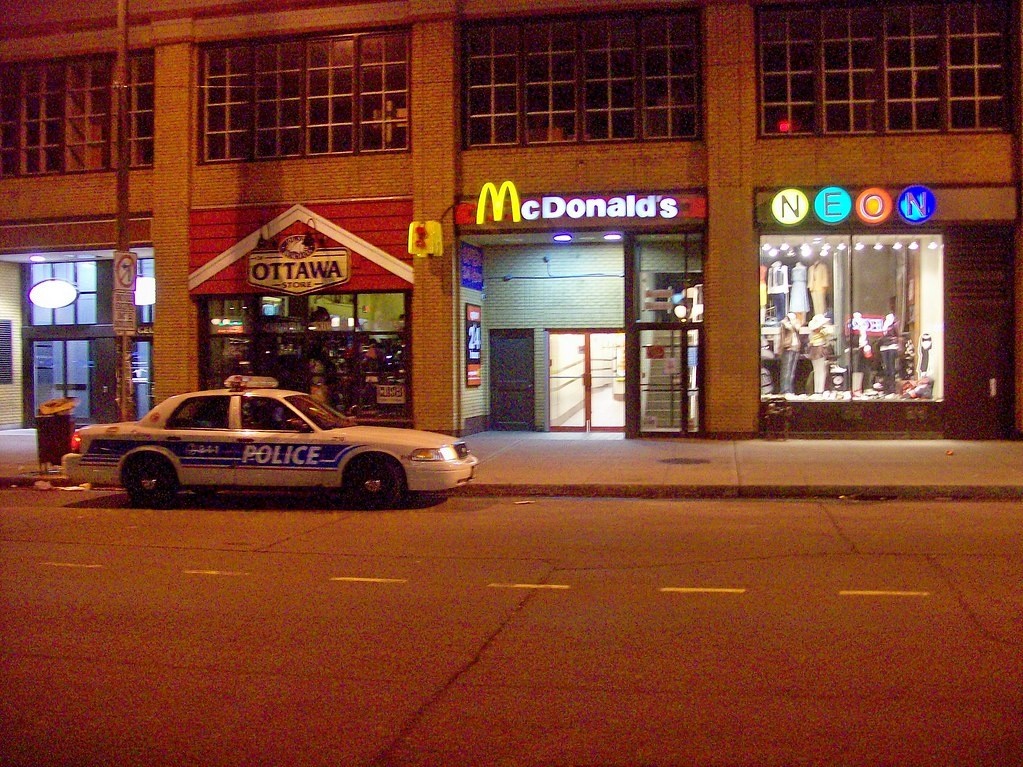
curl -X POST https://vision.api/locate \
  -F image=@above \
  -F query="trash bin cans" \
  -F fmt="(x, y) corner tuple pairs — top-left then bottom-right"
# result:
(764, 397), (789, 440)
(35, 397), (76, 471)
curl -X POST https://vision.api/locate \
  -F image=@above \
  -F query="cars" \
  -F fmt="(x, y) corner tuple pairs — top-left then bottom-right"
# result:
(62, 375), (479, 511)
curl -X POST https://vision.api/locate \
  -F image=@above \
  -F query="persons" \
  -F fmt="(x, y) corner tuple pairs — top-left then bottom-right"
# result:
(878, 313), (901, 398)
(759, 260), (874, 396)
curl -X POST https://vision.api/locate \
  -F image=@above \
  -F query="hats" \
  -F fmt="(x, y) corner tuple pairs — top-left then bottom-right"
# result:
(808, 314), (830, 330)
